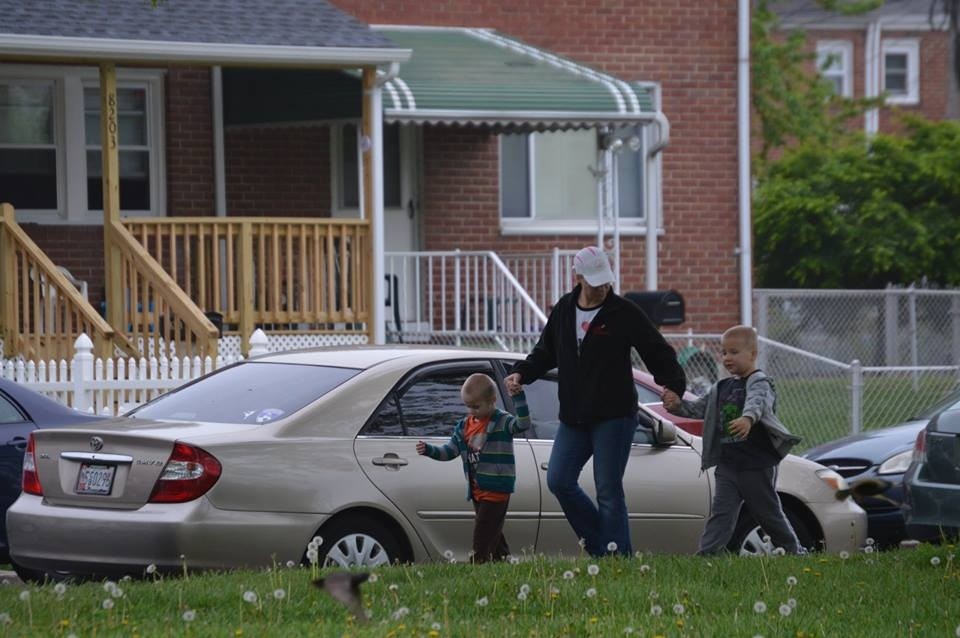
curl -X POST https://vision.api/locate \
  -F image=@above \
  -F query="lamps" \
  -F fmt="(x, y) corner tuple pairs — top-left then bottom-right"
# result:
(595, 129), (642, 155)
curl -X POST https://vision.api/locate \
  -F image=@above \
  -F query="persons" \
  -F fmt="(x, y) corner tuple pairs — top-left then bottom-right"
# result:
(415, 373), (532, 565)
(662, 325), (809, 558)
(504, 246), (688, 560)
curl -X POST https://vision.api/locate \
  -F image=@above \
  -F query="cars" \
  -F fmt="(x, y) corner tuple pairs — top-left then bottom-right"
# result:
(795, 389), (960, 549)
(0, 370), (114, 564)
(902, 397), (960, 546)
(4, 340), (873, 593)
(629, 365), (709, 438)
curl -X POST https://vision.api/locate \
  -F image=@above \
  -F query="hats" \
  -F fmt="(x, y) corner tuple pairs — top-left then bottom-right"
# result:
(573, 247), (615, 287)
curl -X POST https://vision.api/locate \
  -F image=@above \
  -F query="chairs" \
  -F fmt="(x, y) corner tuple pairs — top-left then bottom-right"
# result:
(30, 264), (89, 335)
(321, 236), (409, 344)
(100, 289), (154, 332)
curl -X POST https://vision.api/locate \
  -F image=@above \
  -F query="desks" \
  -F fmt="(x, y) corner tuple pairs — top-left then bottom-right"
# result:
(461, 296), (526, 332)
